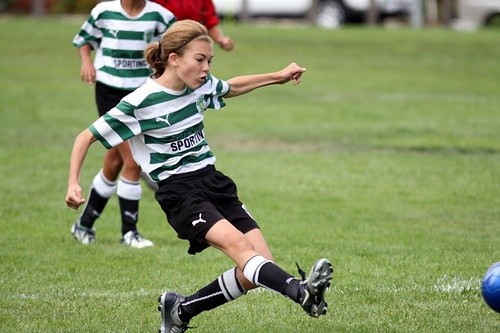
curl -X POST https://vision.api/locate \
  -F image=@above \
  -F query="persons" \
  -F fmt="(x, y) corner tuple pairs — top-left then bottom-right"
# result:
(65, 18), (333, 333)
(306, 0), (500, 33)
(152, 0), (234, 51)
(69, 0), (178, 249)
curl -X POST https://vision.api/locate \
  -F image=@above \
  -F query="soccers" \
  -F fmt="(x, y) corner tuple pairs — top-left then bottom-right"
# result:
(481, 261), (500, 313)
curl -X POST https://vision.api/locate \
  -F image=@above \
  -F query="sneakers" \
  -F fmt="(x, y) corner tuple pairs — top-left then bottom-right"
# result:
(295, 259), (333, 318)
(121, 230), (153, 248)
(158, 291), (197, 333)
(71, 221), (96, 245)
